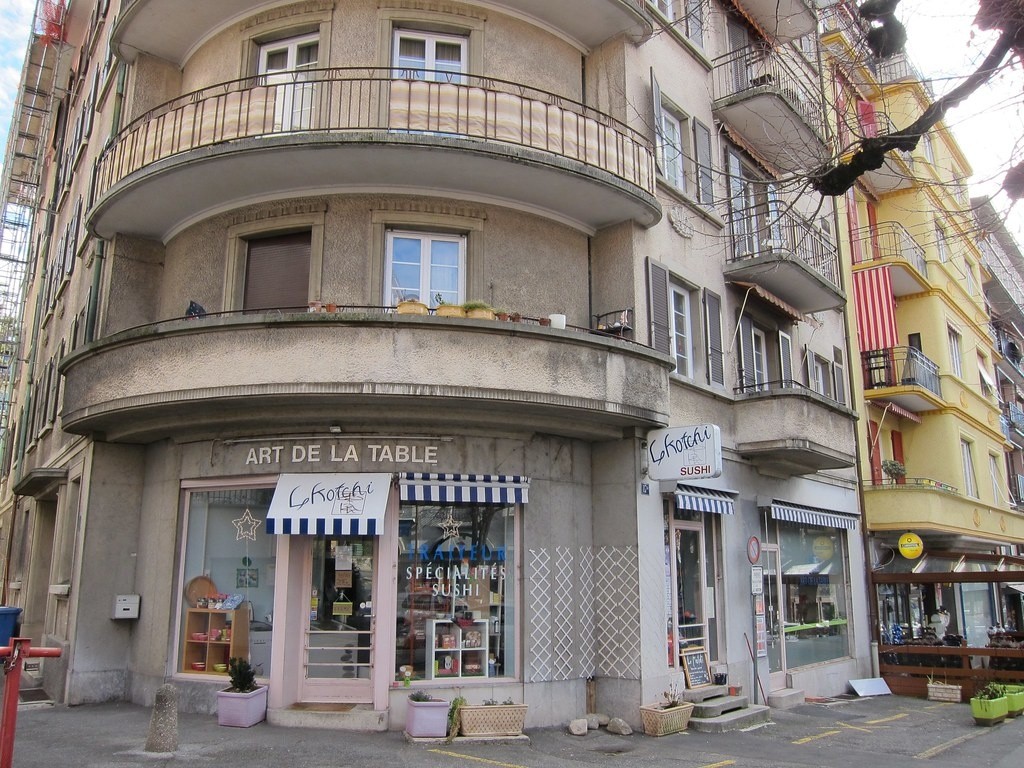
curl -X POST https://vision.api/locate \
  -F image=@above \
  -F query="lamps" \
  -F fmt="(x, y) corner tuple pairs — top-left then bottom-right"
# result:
(329, 423), (342, 433)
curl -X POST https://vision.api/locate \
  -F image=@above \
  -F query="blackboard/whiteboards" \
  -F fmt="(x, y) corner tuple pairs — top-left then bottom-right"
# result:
(684, 648), (711, 687)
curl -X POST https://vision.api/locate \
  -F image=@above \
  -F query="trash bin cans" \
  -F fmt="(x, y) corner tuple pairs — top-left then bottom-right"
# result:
(0, 606), (23, 647)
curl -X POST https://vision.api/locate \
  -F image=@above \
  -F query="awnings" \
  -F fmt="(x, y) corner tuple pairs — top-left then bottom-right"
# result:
(866, 399), (922, 456)
(725, 0), (780, 54)
(838, 64), (867, 102)
(728, 280), (822, 381)
(399, 472), (529, 504)
(857, 177), (879, 201)
(770, 501), (857, 530)
(674, 487), (734, 515)
(721, 123), (783, 181)
(265, 474), (394, 535)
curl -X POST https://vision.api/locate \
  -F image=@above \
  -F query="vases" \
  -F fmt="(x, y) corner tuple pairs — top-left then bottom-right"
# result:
(1001, 685), (1024, 718)
(326, 303), (337, 312)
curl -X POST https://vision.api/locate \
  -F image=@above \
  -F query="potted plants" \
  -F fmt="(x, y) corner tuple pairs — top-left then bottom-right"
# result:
(969, 681), (1008, 726)
(511, 312), (522, 322)
(216, 656), (268, 727)
(435, 696), (528, 746)
(397, 298), (511, 321)
(924, 667), (963, 702)
(539, 317), (551, 327)
(308, 295), (322, 312)
(406, 688), (451, 737)
(640, 681), (696, 734)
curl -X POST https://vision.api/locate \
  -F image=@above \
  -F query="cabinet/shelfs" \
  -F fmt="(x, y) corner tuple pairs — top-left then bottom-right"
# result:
(425, 619), (489, 680)
(181, 607), (250, 675)
(408, 564), (450, 665)
